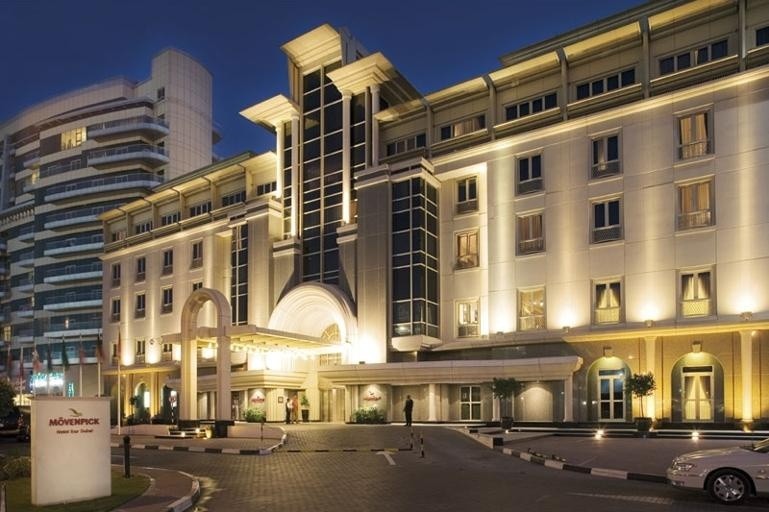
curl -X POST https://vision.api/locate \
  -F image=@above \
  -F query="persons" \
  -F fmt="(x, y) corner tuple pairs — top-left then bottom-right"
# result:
(285, 398), (294, 424)
(402, 395), (414, 427)
(291, 393), (299, 424)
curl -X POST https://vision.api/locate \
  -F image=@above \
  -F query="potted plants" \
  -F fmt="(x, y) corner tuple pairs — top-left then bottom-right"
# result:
(486, 377), (528, 429)
(626, 374), (657, 431)
(300, 394), (309, 422)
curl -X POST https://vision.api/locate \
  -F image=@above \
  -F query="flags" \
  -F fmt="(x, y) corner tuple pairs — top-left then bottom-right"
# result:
(19, 352), (24, 377)
(95, 336), (103, 360)
(6, 353), (13, 380)
(115, 333), (121, 357)
(61, 342), (71, 370)
(47, 348), (53, 373)
(32, 351), (42, 372)
(79, 342), (86, 365)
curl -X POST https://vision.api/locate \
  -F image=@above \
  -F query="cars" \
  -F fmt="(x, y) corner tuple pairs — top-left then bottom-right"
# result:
(665, 438), (769, 504)
